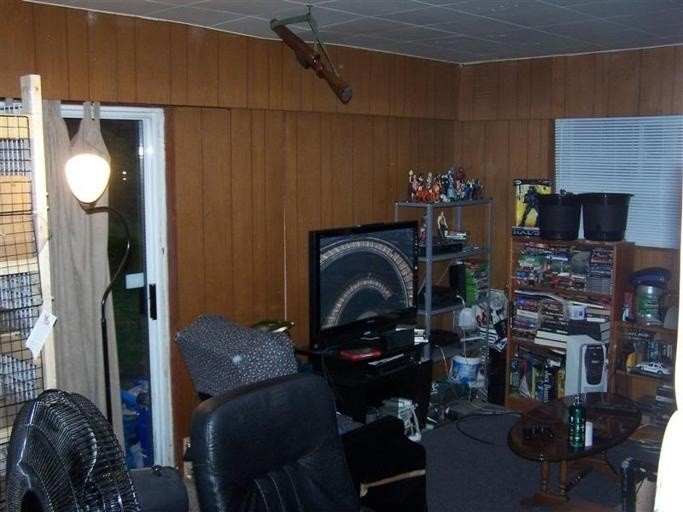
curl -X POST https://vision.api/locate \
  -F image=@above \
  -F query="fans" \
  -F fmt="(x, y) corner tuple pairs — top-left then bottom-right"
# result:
(4, 388), (141, 512)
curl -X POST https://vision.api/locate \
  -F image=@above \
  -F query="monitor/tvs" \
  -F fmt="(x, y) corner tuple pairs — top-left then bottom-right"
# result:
(309, 220), (418, 348)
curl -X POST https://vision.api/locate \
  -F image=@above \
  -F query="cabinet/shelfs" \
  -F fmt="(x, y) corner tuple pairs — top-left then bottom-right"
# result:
(614, 322), (677, 410)
(393, 197), (494, 431)
(296, 329), (460, 434)
(502, 235), (634, 415)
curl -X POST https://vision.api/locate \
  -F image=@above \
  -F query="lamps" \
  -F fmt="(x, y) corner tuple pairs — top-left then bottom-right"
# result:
(58, 9), (114, 204)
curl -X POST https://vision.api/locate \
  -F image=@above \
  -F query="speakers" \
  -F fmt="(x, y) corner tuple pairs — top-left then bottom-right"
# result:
(448, 264), (467, 302)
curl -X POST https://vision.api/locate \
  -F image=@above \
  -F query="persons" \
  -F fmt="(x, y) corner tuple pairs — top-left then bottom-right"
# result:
(408, 167), (485, 203)
(437, 211), (448, 241)
(418, 213), (427, 245)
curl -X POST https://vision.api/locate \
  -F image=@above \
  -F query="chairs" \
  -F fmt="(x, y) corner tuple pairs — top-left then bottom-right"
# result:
(619, 455), (656, 512)
(174, 314), (430, 510)
(189, 370), (360, 510)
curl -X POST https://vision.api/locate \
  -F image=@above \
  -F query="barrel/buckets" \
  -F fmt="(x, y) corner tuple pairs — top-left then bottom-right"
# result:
(532, 194), (582, 241)
(578, 193), (634, 241)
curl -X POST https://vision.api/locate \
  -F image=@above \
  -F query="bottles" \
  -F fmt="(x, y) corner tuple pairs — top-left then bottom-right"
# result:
(568, 393), (586, 452)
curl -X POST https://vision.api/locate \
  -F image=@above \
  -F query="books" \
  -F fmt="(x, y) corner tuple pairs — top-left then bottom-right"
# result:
(618, 331), (673, 362)
(509, 295), (611, 403)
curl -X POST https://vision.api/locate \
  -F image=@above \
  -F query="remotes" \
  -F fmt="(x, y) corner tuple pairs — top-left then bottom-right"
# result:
(596, 400), (638, 415)
